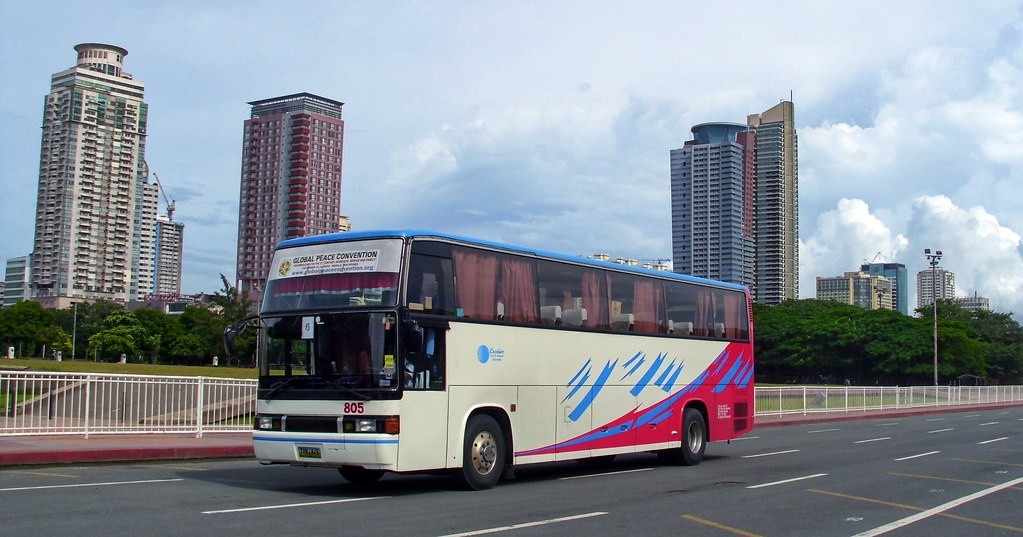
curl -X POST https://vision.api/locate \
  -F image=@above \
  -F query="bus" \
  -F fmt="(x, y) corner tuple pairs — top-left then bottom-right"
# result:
(223, 228), (757, 491)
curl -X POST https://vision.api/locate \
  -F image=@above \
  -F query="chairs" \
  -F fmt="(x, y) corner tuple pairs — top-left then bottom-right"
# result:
(495, 302), (726, 339)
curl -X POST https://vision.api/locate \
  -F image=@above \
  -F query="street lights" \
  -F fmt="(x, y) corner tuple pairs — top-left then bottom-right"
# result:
(925, 248), (943, 386)
